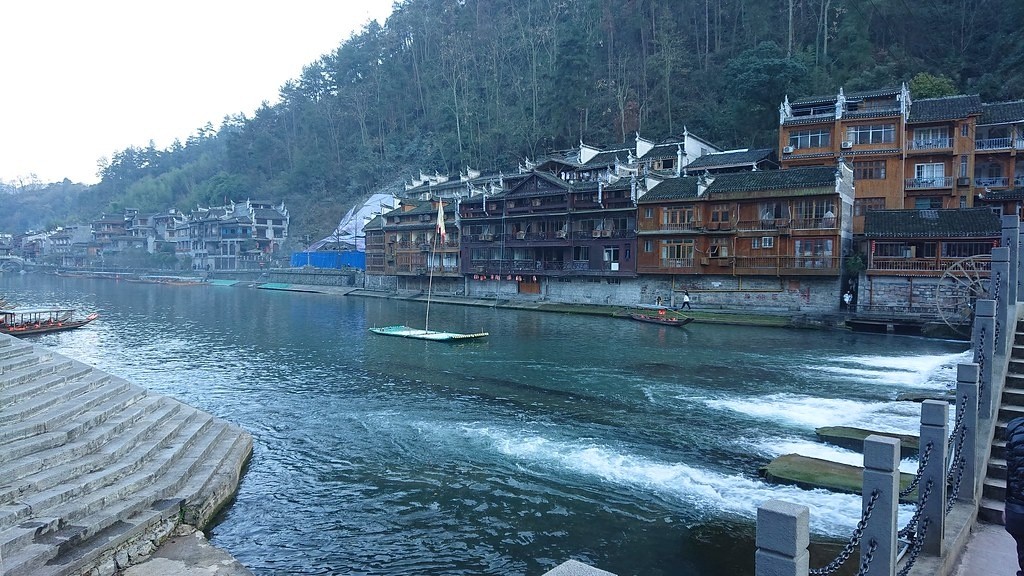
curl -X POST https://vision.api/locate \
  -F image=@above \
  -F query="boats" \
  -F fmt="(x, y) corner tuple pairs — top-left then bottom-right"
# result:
(628, 304), (696, 326)
(0, 306), (99, 336)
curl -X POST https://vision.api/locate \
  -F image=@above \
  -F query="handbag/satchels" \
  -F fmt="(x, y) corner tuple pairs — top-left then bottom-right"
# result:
(1005, 502), (1024, 535)
(684, 295), (689, 302)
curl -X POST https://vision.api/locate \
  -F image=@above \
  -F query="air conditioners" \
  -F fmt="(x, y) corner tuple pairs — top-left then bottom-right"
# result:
(532, 199), (540, 206)
(490, 205), (496, 210)
(602, 230), (611, 238)
(479, 235), (485, 240)
(593, 231), (600, 237)
(762, 237), (773, 248)
(842, 142), (852, 148)
(507, 203), (514, 208)
(958, 179), (969, 185)
(485, 234), (493, 241)
(420, 246), (429, 252)
(517, 231), (524, 239)
(557, 232), (565, 238)
(783, 147), (793, 153)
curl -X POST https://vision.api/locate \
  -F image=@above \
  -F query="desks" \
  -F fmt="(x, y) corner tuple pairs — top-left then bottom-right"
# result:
(926, 143), (937, 149)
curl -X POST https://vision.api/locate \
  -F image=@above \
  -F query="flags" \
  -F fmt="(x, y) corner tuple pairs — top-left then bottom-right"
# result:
(437, 200), (445, 245)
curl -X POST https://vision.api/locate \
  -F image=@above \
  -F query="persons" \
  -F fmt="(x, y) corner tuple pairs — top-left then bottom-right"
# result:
(1004, 417), (1024, 576)
(843, 277), (859, 312)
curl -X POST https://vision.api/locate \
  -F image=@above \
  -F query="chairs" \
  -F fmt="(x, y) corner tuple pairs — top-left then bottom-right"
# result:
(917, 141), (926, 149)
